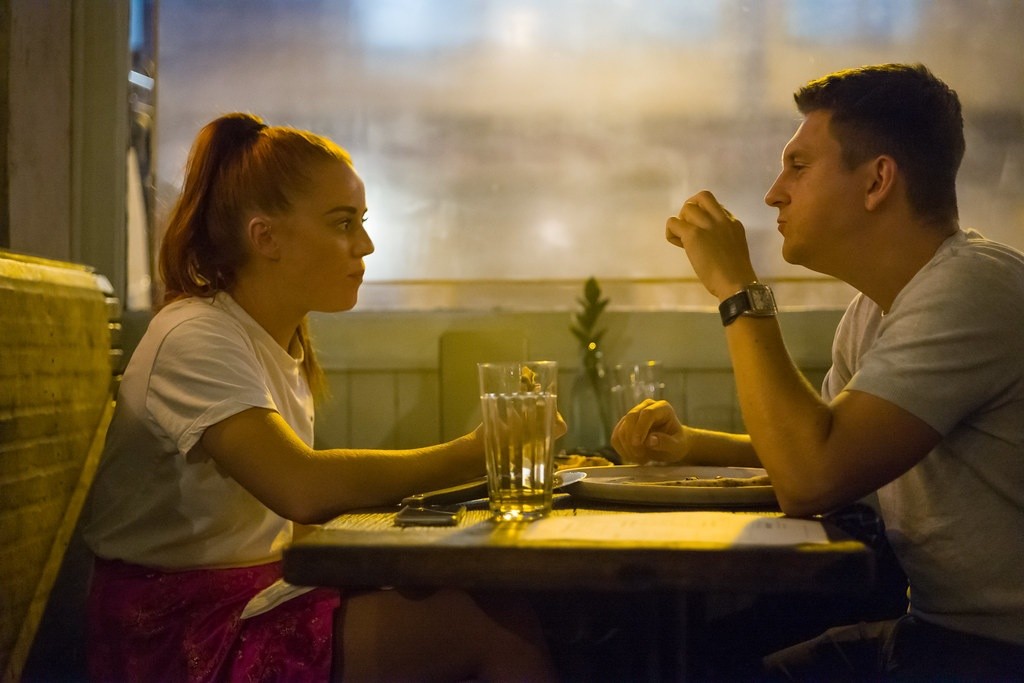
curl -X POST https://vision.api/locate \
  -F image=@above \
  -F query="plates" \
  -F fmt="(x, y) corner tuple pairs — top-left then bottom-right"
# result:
(555, 465), (780, 505)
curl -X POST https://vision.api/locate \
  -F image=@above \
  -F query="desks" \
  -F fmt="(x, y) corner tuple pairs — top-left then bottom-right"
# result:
(283, 458), (878, 683)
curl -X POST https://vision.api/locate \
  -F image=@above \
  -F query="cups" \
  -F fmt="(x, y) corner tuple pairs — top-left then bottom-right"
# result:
(605, 361), (666, 465)
(477, 360), (558, 523)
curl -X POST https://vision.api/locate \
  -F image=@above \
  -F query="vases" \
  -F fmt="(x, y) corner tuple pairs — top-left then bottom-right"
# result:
(574, 374), (610, 450)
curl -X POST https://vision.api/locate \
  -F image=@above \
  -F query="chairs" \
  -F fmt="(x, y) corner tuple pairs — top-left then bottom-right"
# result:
(0, 248), (131, 683)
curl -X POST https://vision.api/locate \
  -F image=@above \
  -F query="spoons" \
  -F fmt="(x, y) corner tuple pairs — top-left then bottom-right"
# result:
(552, 472), (588, 489)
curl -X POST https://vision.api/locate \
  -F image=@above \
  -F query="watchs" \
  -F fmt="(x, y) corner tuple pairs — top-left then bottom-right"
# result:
(719, 282), (778, 326)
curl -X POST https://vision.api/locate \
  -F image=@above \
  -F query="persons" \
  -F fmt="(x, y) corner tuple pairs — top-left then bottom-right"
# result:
(609, 63), (1024, 683)
(81, 112), (567, 683)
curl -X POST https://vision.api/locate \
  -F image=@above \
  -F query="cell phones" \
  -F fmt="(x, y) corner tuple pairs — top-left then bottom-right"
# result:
(394, 501), (465, 528)
